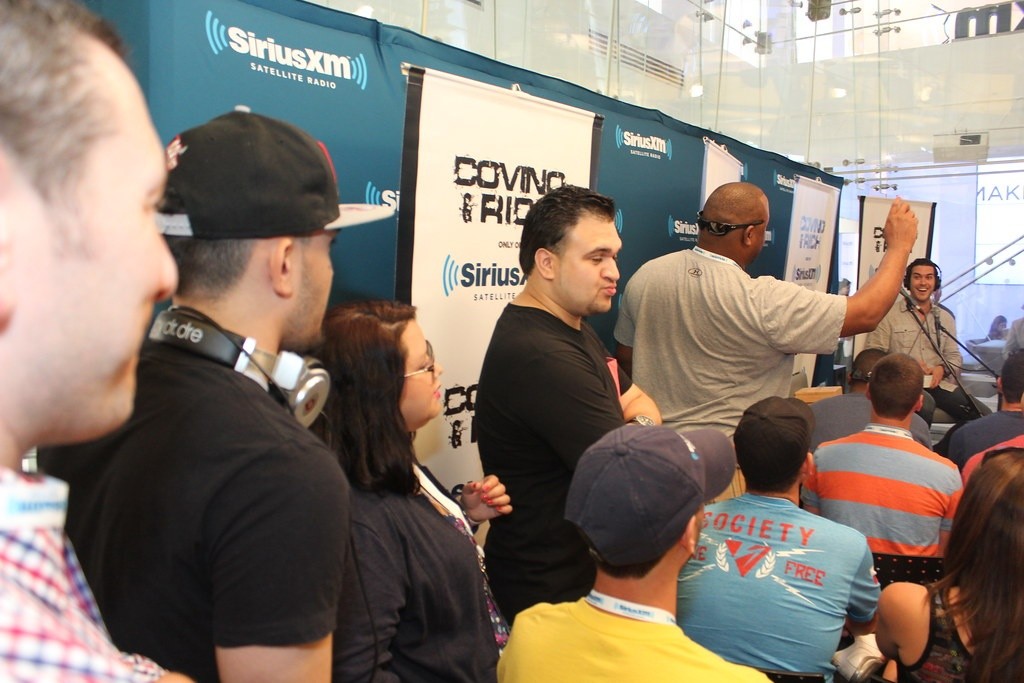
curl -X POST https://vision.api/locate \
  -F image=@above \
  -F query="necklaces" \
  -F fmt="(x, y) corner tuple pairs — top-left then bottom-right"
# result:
(419, 485), (487, 558)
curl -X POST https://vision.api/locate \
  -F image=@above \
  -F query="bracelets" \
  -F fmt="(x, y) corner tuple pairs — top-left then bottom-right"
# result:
(468, 518), (485, 526)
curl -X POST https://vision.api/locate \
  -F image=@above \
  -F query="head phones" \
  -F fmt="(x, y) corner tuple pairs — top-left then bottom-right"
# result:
(903, 259), (942, 293)
(149, 310), (330, 428)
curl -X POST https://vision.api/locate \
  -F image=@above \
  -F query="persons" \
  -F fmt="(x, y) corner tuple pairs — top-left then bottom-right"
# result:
(35, 112), (351, 683)
(948, 349), (1024, 473)
(980, 304), (1024, 360)
(475, 185), (663, 629)
(801, 348), (931, 515)
(615, 181), (918, 505)
(312, 301), (513, 683)
(1, 0), (195, 683)
(835, 279), (851, 364)
(801, 353), (964, 558)
(497, 424), (775, 683)
(863, 258), (992, 455)
(930, 289), (955, 318)
(677, 396), (885, 683)
(875, 445), (1024, 683)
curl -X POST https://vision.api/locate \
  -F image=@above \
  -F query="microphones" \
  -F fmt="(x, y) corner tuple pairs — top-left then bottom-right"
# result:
(899, 287), (924, 314)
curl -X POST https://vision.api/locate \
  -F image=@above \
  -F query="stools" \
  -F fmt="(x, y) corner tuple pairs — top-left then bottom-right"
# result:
(934, 409), (955, 432)
(795, 387), (842, 403)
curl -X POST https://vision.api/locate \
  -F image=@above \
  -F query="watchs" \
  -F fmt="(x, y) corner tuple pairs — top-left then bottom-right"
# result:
(629, 416), (653, 425)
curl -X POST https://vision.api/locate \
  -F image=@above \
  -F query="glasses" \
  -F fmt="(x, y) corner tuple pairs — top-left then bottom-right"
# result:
(697, 209), (764, 236)
(982, 445), (1024, 466)
(403, 340), (434, 379)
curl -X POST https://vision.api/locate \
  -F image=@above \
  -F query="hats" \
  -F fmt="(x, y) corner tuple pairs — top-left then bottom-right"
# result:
(732, 395), (814, 479)
(154, 104), (395, 238)
(564, 424), (735, 568)
